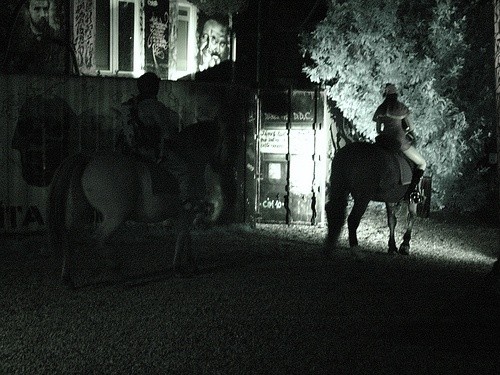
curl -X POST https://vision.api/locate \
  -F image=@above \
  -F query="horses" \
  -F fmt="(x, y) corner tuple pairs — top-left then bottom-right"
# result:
(324, 119), (420, 257)
(45, 117), (226, 290)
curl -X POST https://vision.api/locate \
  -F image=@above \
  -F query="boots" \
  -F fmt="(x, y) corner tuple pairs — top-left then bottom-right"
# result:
(405, 168), (423, 201)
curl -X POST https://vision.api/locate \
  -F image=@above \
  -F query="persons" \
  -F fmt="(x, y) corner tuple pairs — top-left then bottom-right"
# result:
(122, 73), (198, 216)
(7, 0), (76, 73)
(373, 83), (422, 179)
(10, 66), (85, 272)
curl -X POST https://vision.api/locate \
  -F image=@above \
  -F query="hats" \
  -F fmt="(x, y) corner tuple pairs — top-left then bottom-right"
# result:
(134, 73), (158, 95)
(385, 86), (397, 94)
